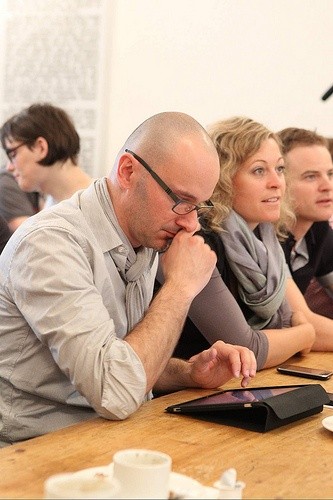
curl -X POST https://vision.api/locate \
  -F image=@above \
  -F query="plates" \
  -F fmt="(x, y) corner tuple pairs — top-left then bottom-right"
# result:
(45, 462), (219, 500)
(321, 415), (333, 433)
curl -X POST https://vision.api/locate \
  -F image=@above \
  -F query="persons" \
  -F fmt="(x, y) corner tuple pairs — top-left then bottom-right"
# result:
(0, 112), (257, 450)
(0, 104), (92, 253)
(147, 116), (333, 368)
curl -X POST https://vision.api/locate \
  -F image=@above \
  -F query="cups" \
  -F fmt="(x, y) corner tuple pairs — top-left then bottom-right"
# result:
(113, 448), (172, 499)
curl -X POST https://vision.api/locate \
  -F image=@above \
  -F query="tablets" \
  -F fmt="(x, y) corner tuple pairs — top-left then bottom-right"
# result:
(166, 385), (309, 414)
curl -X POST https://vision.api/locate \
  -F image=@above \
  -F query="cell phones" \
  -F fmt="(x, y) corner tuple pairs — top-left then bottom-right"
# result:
(277, 364), (333, 380)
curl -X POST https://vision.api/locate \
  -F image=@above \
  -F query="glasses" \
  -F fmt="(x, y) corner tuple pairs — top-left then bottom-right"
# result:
(124, 148), (214, 219)
(7, 142), (28, 162)
